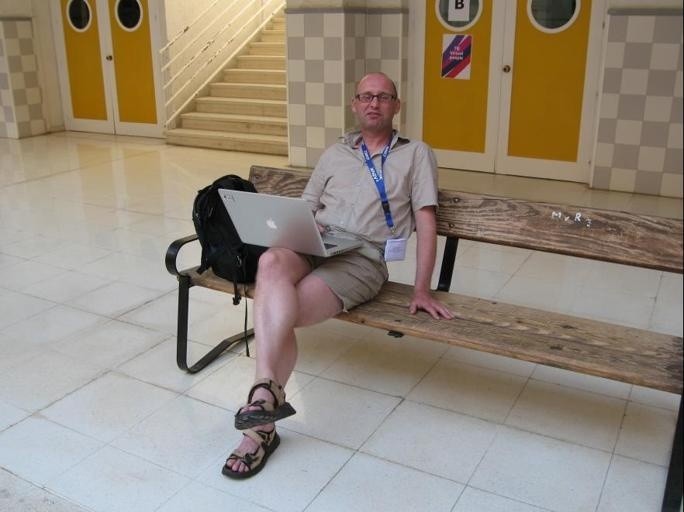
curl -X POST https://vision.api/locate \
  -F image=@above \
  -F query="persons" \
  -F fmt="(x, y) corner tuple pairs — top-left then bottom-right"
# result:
(222, 72), (454, 480)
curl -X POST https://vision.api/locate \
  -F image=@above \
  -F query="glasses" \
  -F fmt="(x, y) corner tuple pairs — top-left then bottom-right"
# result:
(357, 93), (393, 104)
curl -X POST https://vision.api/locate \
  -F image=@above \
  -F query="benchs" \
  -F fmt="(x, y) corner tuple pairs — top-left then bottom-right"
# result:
(166, 166), (683, 511)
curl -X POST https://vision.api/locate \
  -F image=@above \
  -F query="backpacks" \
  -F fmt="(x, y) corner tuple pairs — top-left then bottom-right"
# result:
(192, 174), (269, 286)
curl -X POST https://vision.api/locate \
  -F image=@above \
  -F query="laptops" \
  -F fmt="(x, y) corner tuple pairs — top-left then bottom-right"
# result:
(217, 187), (363, 258)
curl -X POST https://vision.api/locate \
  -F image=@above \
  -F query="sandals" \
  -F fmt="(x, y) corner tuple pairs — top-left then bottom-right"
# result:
(220, 423), (282, 480)
(233, 377), (296, 431)
